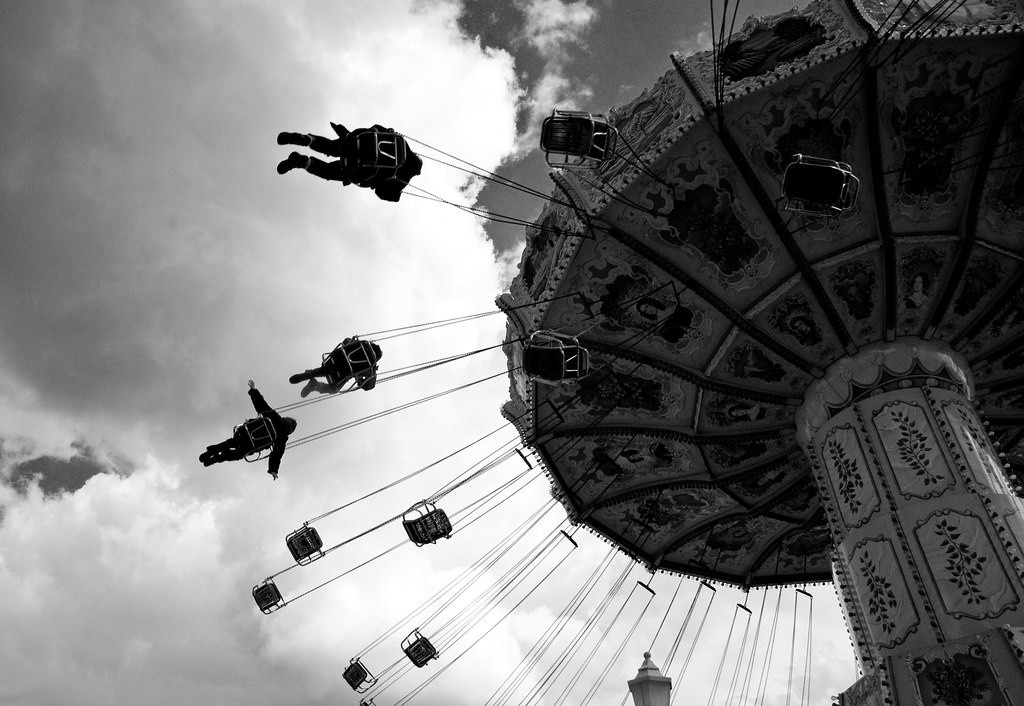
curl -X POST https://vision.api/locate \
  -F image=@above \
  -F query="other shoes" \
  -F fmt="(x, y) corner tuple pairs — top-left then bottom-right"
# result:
(199, 450), (219, 466)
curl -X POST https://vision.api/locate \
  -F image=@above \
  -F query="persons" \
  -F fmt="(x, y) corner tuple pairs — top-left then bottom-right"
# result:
(289, 338), (382, 398)
(198, 378), (298, 481)
(276, 121), (422, 202)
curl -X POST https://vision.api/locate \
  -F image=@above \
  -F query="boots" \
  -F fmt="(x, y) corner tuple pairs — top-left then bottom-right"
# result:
(289, 369), (316, 384)
(301, 378), (323, 397)
(277, 151), (308, 174)
(277, 132), (311, 146)
